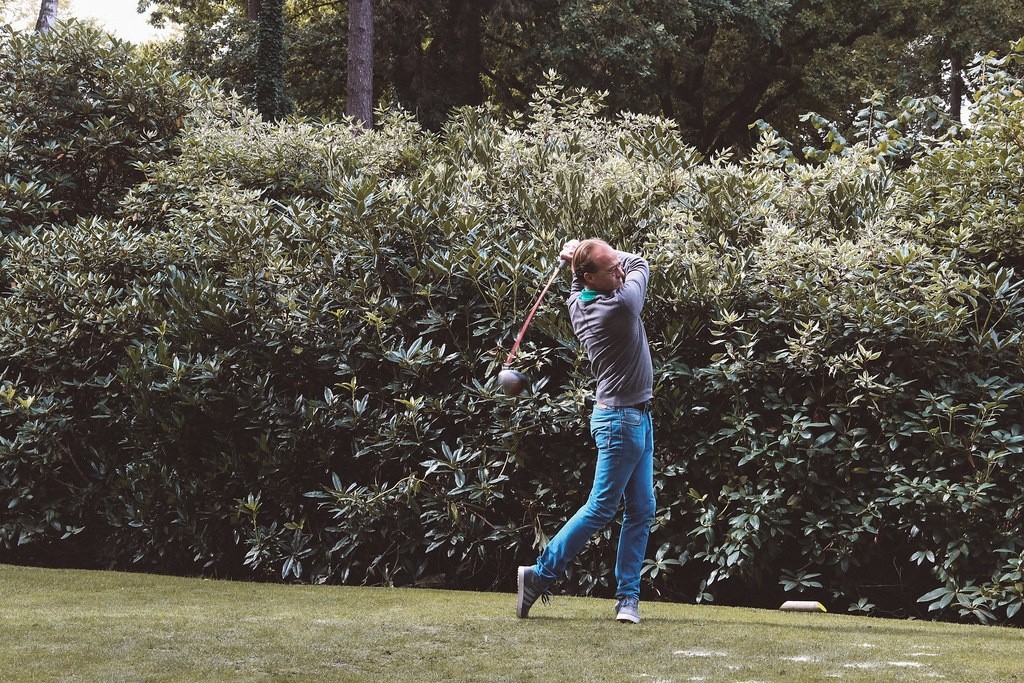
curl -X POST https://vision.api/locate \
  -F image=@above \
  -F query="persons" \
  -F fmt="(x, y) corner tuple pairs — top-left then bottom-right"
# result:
(516, 238), (656, 624)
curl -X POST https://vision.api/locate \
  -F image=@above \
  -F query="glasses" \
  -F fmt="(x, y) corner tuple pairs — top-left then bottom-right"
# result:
(593, 262), (624, 276)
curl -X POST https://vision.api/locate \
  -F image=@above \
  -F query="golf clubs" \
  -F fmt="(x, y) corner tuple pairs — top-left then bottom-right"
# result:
(498, 259), (568, 399)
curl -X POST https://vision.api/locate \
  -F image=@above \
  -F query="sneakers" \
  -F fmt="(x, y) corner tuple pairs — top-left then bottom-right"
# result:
(615, 594), (641, 624)
(516, 566), (554, 619)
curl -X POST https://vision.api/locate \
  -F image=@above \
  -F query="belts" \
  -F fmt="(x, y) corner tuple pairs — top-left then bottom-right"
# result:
(597, 401), (653, 414)
